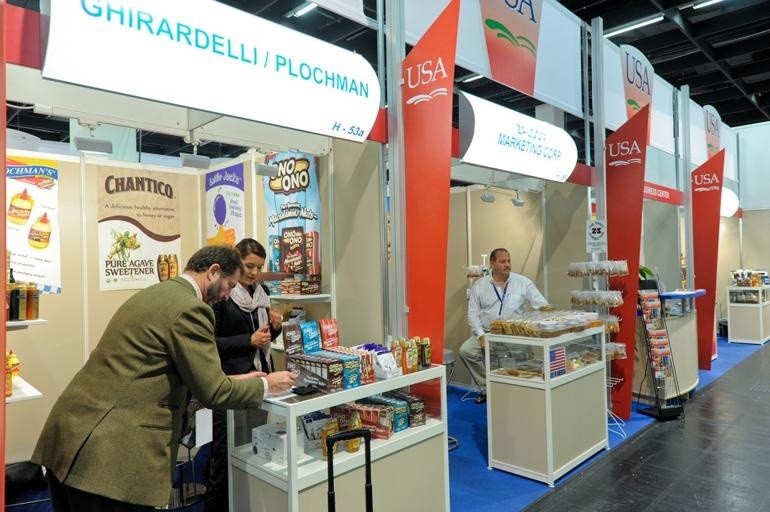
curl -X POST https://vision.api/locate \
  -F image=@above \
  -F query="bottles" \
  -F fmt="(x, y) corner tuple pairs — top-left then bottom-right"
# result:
(8, 269), (40, 320)
(390, 336), (431, 376)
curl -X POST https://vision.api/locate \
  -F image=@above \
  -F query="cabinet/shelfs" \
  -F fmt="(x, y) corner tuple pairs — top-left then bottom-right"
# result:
(270, 293), (331, 352)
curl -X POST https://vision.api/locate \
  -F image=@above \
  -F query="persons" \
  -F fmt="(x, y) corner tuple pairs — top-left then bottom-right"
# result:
(455, 246), (554, 406)
(199, 236), (285, 511)
(27, 244), (300, 512)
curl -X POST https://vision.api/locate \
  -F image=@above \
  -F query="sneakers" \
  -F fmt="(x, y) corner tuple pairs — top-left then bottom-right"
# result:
(475, 391), (487, 404)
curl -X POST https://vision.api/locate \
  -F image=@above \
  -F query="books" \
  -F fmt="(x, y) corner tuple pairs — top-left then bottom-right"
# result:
(639, 289), (673, 380)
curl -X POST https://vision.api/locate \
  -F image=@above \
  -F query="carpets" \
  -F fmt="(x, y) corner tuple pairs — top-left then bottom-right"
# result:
(447, 335), (762, 511)
